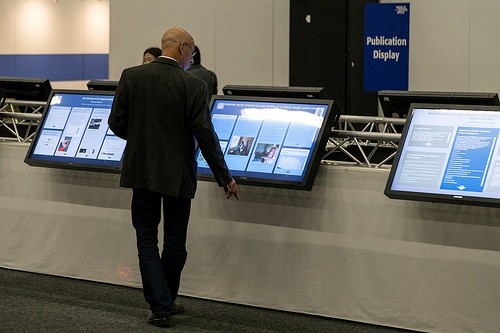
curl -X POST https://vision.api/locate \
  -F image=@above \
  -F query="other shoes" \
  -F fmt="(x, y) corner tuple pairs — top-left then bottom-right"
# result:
(157, 302), (185, 314)
(147, 311), (174, 328)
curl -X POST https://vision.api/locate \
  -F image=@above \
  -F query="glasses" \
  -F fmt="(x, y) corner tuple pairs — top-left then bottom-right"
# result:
(184, 41), (197, 57)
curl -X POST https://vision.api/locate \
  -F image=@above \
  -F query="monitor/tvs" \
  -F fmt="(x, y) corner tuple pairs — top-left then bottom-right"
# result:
(194, 95), (337, 191)
(87, 79), (119, 91)
(222, 85), (324, 100)
(0, 76), (52, 139)
(378, 90), (500, 134)
(384, 103), (500, 207)
(24, 89), (127, 174)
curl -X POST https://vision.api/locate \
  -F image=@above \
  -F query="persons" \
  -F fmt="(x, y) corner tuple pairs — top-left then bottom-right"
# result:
(58, 140), (69, 151)
(253, 144), (279, 163)
(183, 46), (217, 104)
(142, 47), (162, 64)
(107, 27), (239, 329)
(230, 137), (250, 155)
